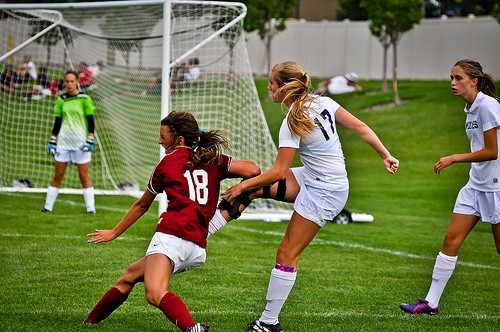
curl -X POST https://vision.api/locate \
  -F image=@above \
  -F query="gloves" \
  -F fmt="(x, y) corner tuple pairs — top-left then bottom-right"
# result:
(80, 141), (94, 152)
(47, 144), (57, 155)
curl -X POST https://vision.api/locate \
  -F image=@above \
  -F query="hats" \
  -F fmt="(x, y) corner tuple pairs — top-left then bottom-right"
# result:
(345, 72), (359, 82)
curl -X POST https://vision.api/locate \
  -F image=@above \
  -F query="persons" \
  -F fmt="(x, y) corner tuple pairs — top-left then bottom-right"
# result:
(79, 110), (261, 332)
(397, 58), (500, 316)
(42, 70), (96, 215)
(206, 60), (401, 332)
(316, 72), (364, 97)
(141, 57), (201, 96)
(0, 54), (106, 103)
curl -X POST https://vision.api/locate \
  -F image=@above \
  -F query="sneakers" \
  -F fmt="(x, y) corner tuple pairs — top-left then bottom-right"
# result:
(244, 320), (283, 332)
(185, 322), (210, 332)
(400, 298), (440, 315)
